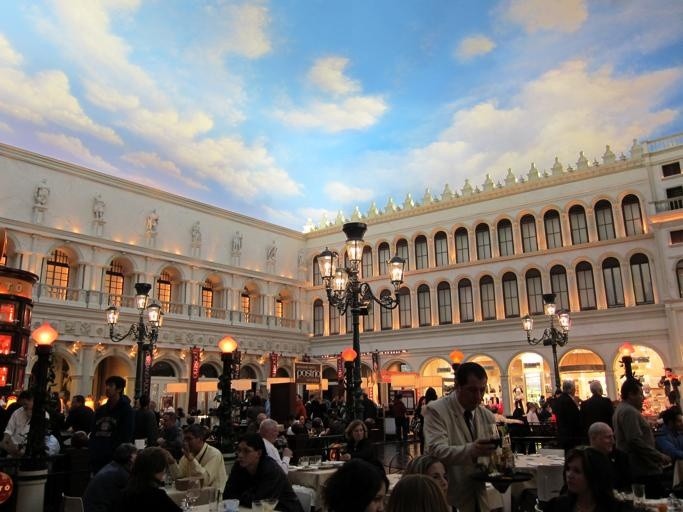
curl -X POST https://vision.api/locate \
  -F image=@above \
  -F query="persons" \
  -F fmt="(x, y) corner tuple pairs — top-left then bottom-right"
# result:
(146, 208), (158, 232)
(34, 177), (50, 205)
(1, 364), (683, 512)
(94, 193), (105, 220)
(267, 241), (278, 262)
(192, 219), (202, 242)
(231, 231), (243, 255)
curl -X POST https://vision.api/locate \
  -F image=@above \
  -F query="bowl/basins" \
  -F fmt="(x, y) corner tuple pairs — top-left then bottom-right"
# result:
(222, 499), (240, 511)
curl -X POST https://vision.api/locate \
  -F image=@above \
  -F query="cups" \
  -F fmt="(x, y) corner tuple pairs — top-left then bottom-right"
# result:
(252, 502), (264, 512)
(260, 499), (279, 512)
(134, 438), (146, 450)
(207, 488), (223, 512)
(633, 484), (647, 507)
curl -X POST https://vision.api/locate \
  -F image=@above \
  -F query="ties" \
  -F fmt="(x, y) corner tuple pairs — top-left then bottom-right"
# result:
(463, 410), (477, 441)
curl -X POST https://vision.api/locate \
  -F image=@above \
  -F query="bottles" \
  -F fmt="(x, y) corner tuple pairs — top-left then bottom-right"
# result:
(501, 432), (514, 477)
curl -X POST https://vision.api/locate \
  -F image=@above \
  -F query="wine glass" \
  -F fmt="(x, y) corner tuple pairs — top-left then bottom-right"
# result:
(185, 479), (201, 512)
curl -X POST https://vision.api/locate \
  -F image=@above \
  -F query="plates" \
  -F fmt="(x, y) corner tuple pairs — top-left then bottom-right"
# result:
(295, 456), (345, 471)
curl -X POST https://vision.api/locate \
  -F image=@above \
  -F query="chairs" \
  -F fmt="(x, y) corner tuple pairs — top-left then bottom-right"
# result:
(0, 397), (683, 512)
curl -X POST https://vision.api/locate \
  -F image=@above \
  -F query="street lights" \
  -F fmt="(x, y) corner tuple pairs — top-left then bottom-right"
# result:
(105, 282), (165, 411)
(27, 321), (58, 472)
(446, 348), (464, 383)
(337, 348), (361, 418)
(315, 222), (406, 425)
(522, 288), (572, 389)
(217, 336), (239, 451)
(619, 341), (639, 381)
